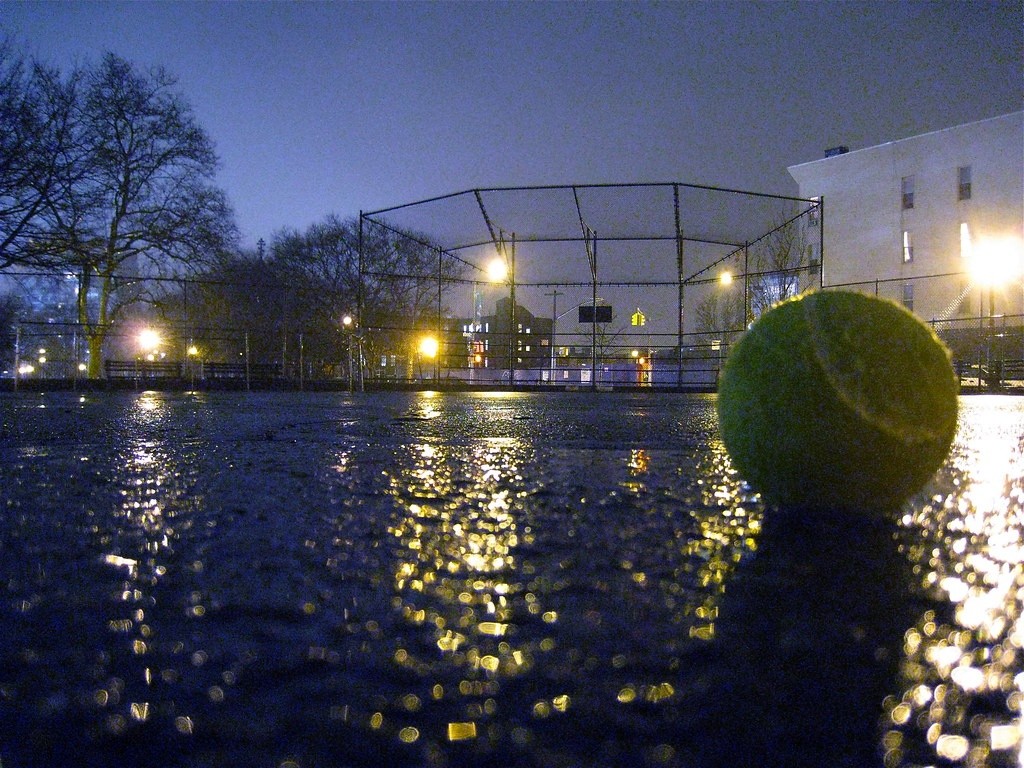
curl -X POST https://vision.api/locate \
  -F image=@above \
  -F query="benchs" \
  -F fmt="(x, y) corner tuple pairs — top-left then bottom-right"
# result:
(201, 361), (284, 393)
(993, 358), (1024, 386)
(104, 360), (185, 391)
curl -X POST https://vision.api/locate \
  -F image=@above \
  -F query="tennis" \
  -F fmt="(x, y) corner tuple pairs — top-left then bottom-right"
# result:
(716, 289), (960, 512)
